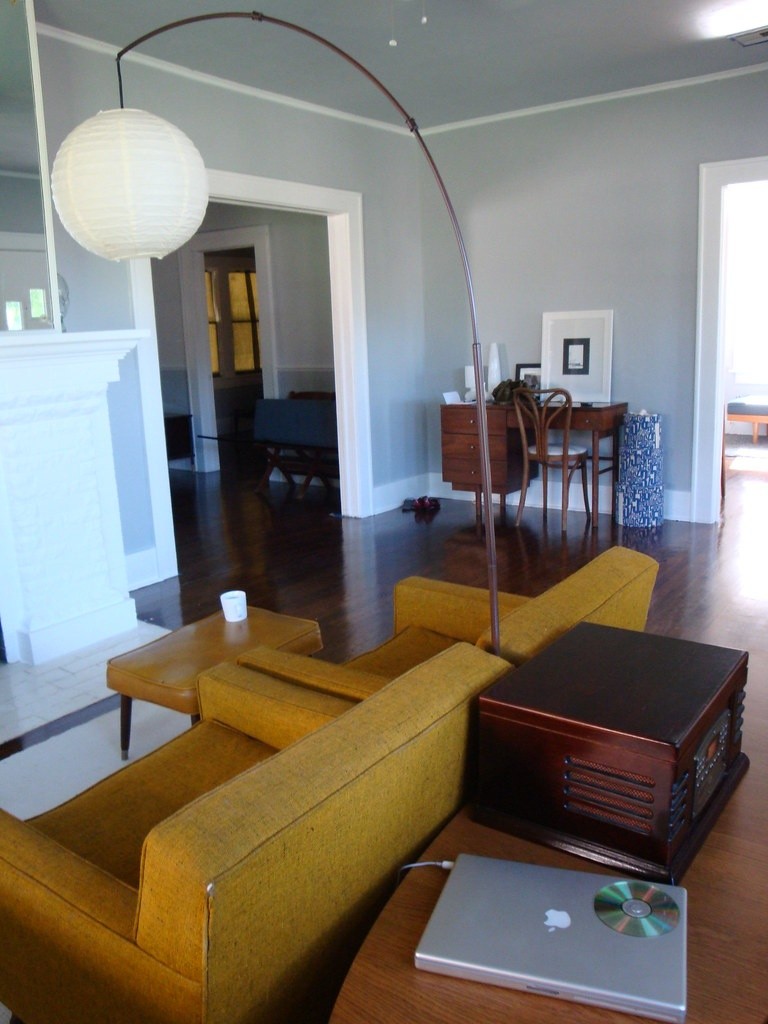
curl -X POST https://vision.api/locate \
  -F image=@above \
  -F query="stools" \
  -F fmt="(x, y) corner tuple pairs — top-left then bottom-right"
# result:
(727, 394), (768, 444)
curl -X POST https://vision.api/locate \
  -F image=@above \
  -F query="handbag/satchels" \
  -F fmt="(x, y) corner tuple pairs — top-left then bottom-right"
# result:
(492, 379), (529, 402)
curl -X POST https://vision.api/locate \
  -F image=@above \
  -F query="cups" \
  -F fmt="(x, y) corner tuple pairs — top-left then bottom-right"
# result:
(220, 590), (247, 622)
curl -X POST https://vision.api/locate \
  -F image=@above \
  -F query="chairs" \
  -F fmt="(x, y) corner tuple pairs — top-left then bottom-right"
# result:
(512, 388), (590, 531)
(254, 391), (334, 501)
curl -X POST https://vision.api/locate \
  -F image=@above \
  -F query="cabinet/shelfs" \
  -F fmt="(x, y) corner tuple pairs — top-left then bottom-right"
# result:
(164, 413), (196, 466)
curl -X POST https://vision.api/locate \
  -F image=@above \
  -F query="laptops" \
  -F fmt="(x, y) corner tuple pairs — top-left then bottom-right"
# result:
(415, 852), (688, 1024)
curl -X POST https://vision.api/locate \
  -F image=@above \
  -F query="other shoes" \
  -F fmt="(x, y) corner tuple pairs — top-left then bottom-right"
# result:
(402, 499), (415, 511)
(415, 496), (440, 513)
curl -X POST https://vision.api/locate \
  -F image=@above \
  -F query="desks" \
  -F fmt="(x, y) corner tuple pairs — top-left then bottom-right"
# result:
(329, 653), (768, 1024)
(196, 433), (340, 506)
(439, 402), (628, 528)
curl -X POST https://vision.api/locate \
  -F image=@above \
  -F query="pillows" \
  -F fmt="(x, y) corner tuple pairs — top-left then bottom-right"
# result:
(250, 397), (337, 448)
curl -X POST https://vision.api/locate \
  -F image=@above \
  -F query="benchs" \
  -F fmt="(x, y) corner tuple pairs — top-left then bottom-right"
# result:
(107, 605), (324, 761)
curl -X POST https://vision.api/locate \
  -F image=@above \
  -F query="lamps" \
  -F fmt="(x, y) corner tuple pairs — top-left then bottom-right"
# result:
(52, 12), (500, 658)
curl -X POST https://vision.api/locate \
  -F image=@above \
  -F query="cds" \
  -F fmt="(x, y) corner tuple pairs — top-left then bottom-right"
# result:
(594, 881), (680, 938)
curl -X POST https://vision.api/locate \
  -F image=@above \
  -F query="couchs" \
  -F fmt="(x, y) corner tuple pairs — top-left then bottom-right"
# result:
(238, 545), (660, 742)
(0, 642), (514, 1024)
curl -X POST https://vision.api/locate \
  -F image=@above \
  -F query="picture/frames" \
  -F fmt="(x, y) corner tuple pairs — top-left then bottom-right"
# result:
(515, 364), (541, 403)
(540, 309), (614, 403)
